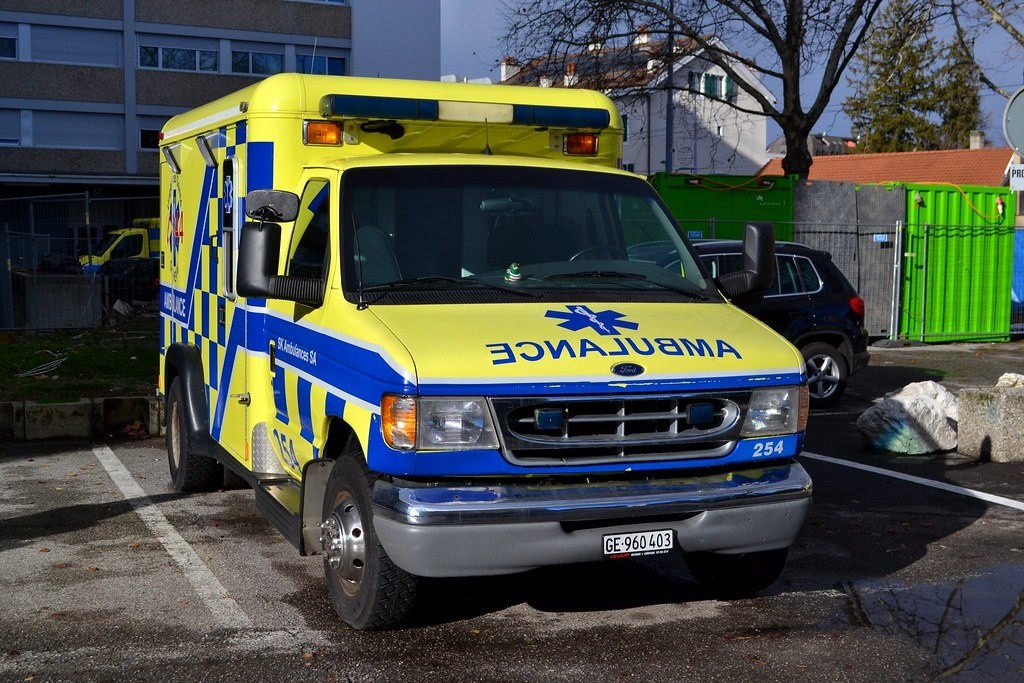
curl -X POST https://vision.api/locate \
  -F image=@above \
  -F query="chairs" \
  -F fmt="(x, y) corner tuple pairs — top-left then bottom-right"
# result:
(486, 214), (571, 270)
(353, 226), (404, 286)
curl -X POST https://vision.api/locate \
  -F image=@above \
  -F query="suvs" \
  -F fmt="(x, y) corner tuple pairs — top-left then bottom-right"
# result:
(627, 238), (869, 409)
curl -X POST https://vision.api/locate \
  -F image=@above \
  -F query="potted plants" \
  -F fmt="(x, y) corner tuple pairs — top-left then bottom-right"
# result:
(9, 252), (102, 329)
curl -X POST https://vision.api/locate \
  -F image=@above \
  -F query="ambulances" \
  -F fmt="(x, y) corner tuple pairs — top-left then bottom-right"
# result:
(80, 218), (163, 270)
(155, 71), (814, 631)
